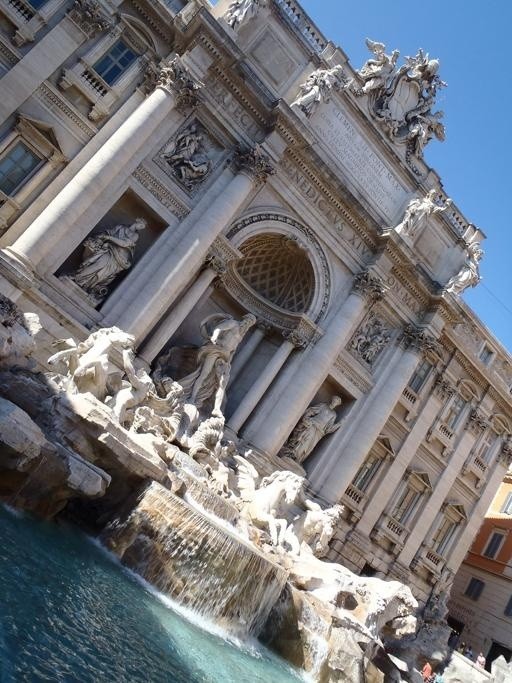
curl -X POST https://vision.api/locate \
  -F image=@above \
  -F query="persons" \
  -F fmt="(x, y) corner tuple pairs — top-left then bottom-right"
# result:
(422, 661), (445, 683)
(443, 241), (486, 296)
(401, 189), (454, 240)
(475, 651), (485, 670)
(358, 49), (401, 94)
(165, 133), (204, 168)
(406, 110), (447, 158)
(178, 312), (257, 421)
(222, 0), (266, 33)
(280, 394), (342, 465)
(455, 641), (467, 655)
(353, 324), (379, 351)
(69, 216), (149, 300)
(293, 64), (342, 120)
(179, 158), (213, 183)
(464, 645), (474, 658)
(362, 328), (387, 363)
